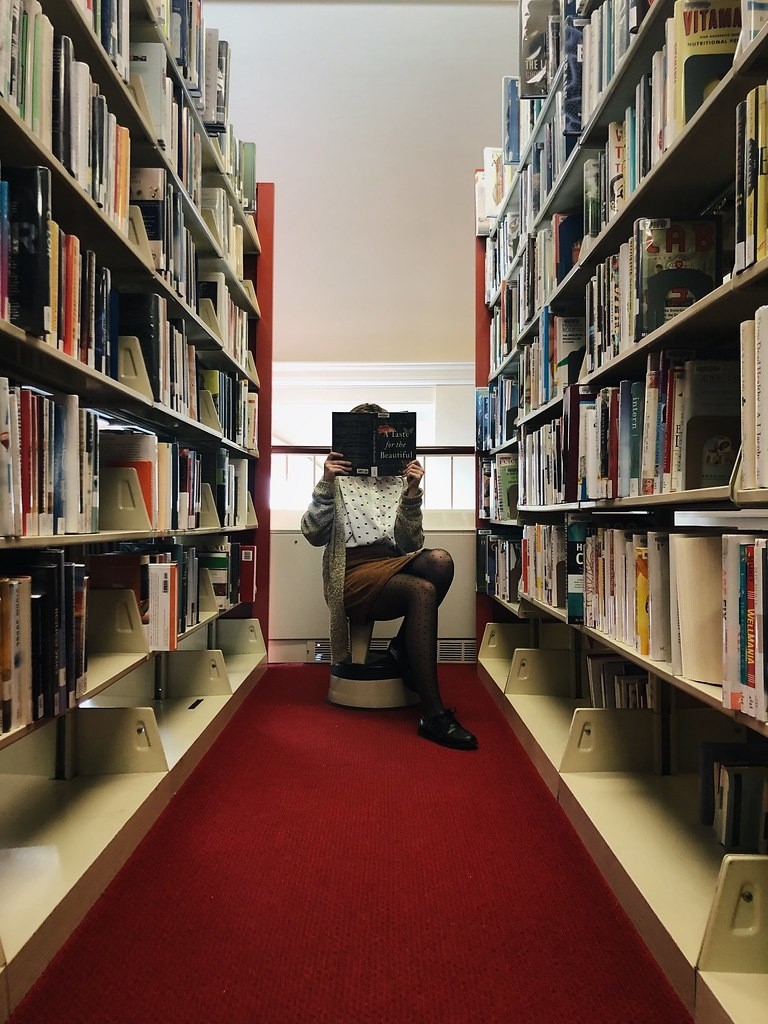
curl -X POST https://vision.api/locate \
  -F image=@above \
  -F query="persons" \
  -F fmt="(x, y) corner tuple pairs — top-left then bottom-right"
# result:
(298, 402), (479, 751)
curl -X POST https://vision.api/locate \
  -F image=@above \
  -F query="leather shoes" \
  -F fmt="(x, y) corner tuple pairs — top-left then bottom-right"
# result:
(385, 637), (417, 693)
(418, 704), (478, 746)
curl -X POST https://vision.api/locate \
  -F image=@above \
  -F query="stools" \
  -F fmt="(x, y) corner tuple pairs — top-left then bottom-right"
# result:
(328, 612), (418, 709)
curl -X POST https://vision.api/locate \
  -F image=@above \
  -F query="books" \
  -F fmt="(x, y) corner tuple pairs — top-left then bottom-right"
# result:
(0, 0), (266, 735)
(330, 412), (418, 478)
(479, 0), (768, 856)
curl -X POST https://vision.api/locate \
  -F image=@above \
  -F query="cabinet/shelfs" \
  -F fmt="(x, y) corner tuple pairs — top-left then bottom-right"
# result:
(474, 0), (768, 1024)
(0, 0), (273, 1024)
